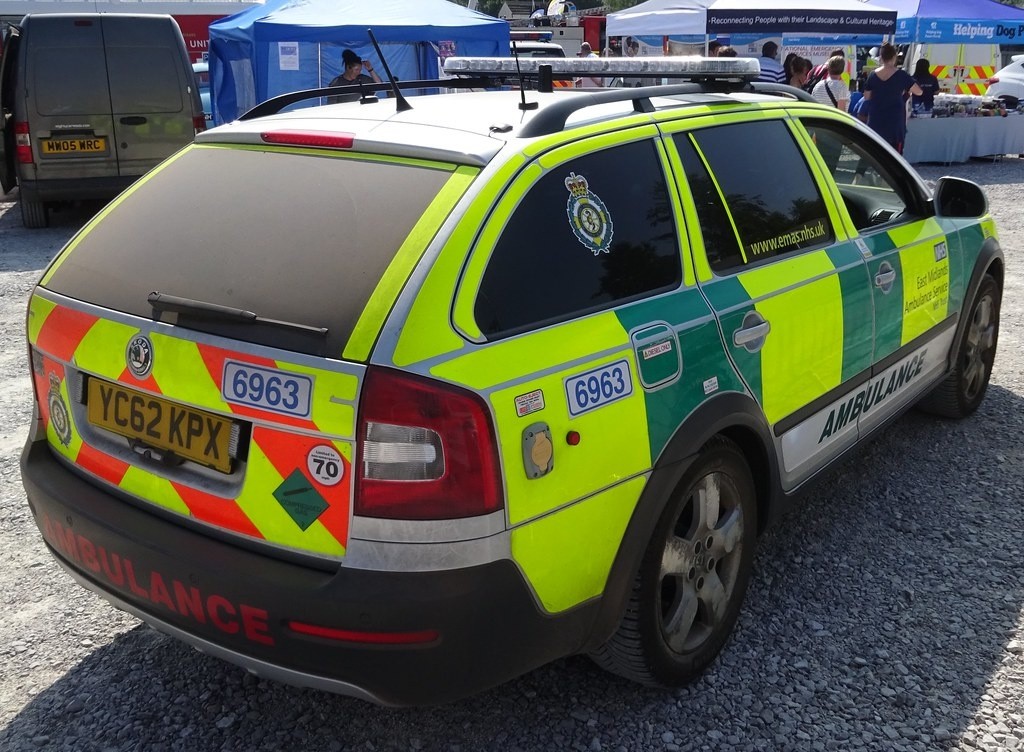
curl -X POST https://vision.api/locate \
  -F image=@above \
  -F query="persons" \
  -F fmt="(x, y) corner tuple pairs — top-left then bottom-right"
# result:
(576, 42), (603, 87)
(707, 42), (741, 83)
(602, 39), (622, 86)
(910, 59), (940, 118)
(756, 41), (786, 83)
(781, 54), (813, 98)
(811, 55), (851, 176)
(852, 43), (922, 186)
(803, 50), (845, 93)
(327, 50), (381, 104)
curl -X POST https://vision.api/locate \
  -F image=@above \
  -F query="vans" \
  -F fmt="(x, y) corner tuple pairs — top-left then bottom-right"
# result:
(0, 11), (210, 230)
(902, 39), (1001, 96)
(507, 33), (578, 91)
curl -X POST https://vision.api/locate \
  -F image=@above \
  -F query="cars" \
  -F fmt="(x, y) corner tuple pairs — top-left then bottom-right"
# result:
(983, 54), (1024, 101)
(930, 100), (950, 118)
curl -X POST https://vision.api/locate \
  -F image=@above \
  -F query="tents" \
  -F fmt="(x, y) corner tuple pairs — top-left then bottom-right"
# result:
(783, 0), (1024, 77)
(606, 0), (898, 58)
(208, 0), (511, 128)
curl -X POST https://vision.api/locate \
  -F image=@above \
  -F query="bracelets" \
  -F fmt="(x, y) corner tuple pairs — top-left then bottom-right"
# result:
(368, 68), (373, 72)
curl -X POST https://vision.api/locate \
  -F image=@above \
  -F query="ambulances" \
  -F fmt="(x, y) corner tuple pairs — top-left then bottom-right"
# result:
(18, 28), (1005, 710)
